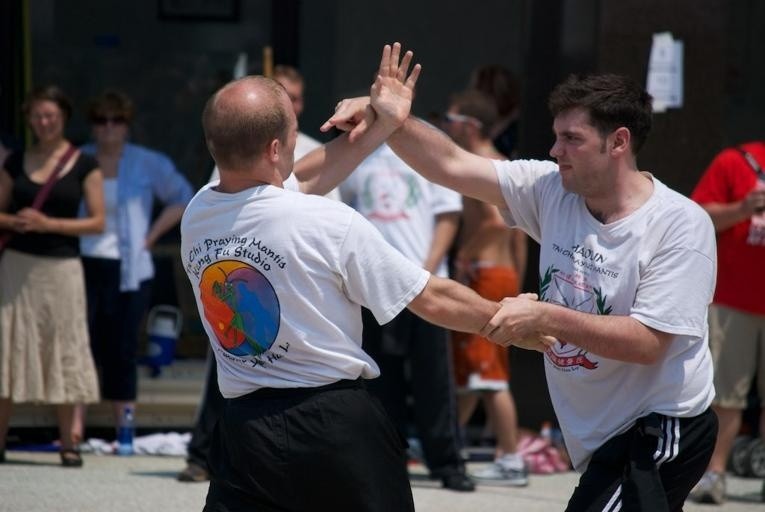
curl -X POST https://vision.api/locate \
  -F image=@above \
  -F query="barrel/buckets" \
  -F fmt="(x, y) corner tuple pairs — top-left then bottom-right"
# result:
(145, 305), (184, 366)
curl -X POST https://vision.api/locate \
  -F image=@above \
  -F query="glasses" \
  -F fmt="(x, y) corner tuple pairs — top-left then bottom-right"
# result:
(91, 114), (126, 126)
(442, 113), (484, 132)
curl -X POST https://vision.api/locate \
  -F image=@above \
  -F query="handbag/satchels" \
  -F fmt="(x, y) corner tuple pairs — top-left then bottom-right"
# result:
(494, 425), (565, 474)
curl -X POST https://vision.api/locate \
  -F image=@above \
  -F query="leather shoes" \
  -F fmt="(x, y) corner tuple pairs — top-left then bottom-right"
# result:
(442, 472), (473, 490)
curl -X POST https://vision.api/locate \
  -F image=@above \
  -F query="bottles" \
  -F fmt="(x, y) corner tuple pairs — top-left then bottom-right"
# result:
(116, 406), (134, 456)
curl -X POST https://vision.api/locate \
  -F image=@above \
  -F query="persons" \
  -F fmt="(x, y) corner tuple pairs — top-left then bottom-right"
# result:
(0, 82), (105, 467)
(180, 41), (555, 512)
(691, 141), (765, 505)
(53, 90), (194, 456)
(321, 74), (718, 512)
(334, 70), (477, 490)
(440, 88), (530, 487)
(176, 63), (340, 483)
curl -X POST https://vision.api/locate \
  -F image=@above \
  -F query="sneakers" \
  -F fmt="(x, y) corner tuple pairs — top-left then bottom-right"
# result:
(471, 464), (528, 487)
(689, 469), (725, 503)
(179, 462), (207, 482)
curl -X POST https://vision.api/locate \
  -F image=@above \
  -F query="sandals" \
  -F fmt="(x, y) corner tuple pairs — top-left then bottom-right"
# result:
(61, 447), (82, 466)
